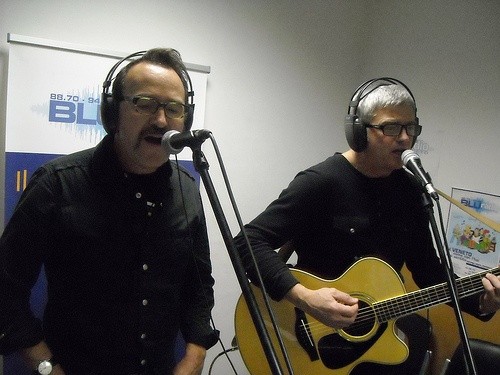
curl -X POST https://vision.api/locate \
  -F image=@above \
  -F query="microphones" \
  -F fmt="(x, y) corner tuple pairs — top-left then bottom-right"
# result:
(401, 149), (439, 201)
(161, 129), (211, 154)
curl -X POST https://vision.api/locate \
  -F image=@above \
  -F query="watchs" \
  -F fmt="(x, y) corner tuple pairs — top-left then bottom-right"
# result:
(30, 356), (58, 375)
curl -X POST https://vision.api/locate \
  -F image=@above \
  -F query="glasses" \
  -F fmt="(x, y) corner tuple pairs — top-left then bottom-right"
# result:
(364, 122), (423, 137)
(115, 95), (192, 121)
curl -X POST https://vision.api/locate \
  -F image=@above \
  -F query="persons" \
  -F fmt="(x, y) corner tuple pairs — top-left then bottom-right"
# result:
(235, 87), (500, 375)
(0, 47), (220, 375)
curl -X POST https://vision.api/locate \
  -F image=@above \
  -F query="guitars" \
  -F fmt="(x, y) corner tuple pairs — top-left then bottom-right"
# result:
(234, 256), (500, 375)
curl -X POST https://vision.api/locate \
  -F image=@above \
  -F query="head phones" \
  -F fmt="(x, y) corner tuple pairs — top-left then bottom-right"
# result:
(101, 51), (195, 134)
(344, 77), (419, 152)
